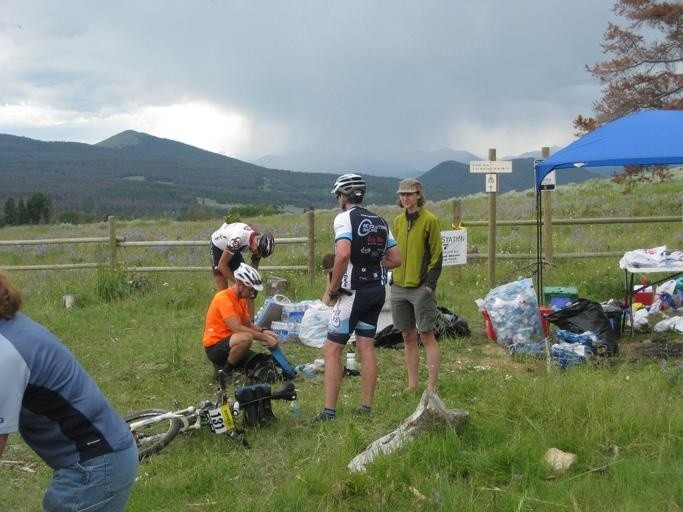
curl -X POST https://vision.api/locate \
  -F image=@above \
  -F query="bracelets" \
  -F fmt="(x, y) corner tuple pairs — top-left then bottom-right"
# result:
(325, 288), (338, 298)
(260, 328), (271, 334)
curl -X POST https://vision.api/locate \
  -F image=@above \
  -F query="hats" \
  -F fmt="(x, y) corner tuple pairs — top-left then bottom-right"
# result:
(396, 178), (421, 193)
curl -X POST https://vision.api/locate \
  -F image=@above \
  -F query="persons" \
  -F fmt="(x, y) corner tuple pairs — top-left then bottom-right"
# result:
(203, 263), (281, 385)
(1, 269), (140, 512)
(320, 253), (339, 309)
(209, 222), (276, 325)
(389, 178), (444, 399)
(307, 174), (403, 425)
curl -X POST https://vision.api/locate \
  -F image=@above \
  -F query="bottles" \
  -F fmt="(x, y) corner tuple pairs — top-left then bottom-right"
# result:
(346, 349), (357, 375)
(290, 396), (300, 417)
(270, 299), (322, 342)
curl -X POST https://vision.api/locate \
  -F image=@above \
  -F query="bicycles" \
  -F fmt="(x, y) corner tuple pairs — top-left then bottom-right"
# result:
(121, 367), (298, 462)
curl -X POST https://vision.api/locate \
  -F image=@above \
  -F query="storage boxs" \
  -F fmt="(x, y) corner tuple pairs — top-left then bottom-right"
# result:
(633, 284), (652, 305)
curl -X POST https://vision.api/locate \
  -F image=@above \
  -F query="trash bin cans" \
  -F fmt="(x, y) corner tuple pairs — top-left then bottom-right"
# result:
(603, 311), (623, 340)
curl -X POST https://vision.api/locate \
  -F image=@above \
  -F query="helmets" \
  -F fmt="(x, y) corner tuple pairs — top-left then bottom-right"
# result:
(331, 174), (366, 195)
(233, 262), (263, 291)
(257, 232), (274, 257)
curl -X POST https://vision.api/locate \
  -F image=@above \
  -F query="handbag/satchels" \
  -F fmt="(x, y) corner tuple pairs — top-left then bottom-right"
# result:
(299, 304), (335, 348)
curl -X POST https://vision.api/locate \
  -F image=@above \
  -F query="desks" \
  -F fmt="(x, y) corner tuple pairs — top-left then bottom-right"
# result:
(620, 265), (682, 338)
